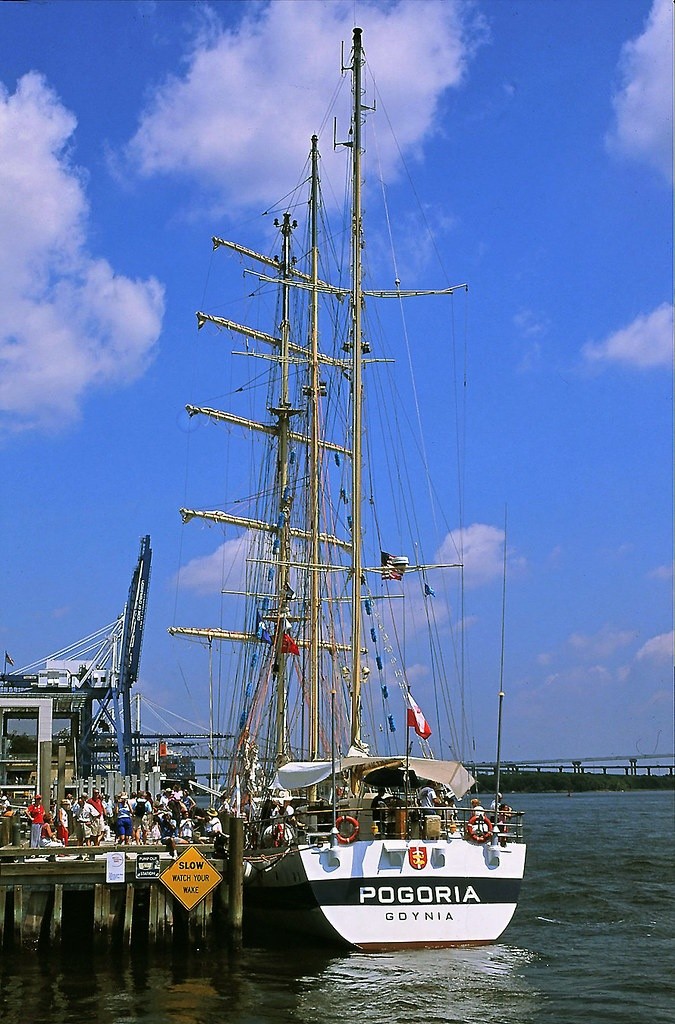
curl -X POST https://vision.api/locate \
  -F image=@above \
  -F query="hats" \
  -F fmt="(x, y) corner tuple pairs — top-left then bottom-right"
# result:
(35, 795), (41, 799)
(207, 808), (218, 817)
(166, 788), (172, 792)
(378, 788), (387, 793)
(1, 796), (7, 800)
(60, 800), (69, 805)
(121, 795), (127, 799)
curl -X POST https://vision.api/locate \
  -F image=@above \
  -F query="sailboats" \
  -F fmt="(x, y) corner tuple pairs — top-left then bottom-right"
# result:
(172, 25), (532, 958)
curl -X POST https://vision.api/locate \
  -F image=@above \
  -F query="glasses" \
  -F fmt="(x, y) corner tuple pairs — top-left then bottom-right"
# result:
(95, 792), (99, 794)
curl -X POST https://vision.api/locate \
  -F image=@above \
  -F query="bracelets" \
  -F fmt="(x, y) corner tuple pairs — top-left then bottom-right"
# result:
(91, 820), (94, 823)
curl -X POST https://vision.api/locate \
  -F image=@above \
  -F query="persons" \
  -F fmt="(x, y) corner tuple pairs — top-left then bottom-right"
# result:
(2, 796), (14, 817)
(471, 798), (486, 823)
(40, 814), (63, 862)
(57, 799), (70, 857)
(261, 799), (294, 825)
(416, 781), (439, 815)
(370, 788), (387, 832)
(74, 798), (100, 861)
(205, 808), (222, 836)
(443, 799), (454, 821)
(24, 795), (45, 858)
(72, 785), (195, 846)
(489, 793), (512, 833)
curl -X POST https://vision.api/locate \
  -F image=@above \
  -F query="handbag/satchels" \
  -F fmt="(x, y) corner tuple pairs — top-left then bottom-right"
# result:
(53, 812), (61, 826)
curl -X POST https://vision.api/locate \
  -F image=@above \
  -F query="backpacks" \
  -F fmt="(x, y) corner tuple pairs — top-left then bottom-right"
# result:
(135, 800), (148, 816)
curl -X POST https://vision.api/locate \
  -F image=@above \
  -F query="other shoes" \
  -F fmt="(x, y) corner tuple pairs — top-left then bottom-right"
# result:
(74, 857), (83, 860)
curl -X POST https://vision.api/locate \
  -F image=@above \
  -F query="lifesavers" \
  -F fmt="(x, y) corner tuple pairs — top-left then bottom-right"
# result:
(467, 814), (494, 842)
(274, 825), (283, 848)
(335, 816), (360, 844)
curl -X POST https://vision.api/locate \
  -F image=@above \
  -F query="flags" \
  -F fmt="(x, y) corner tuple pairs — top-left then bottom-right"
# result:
(5, 653), (15, 667)
(406, 692), (432, 741)
(381, 551), (402, 581)
(281, 634), (300, 656)
(255, 610), (271, 644)
